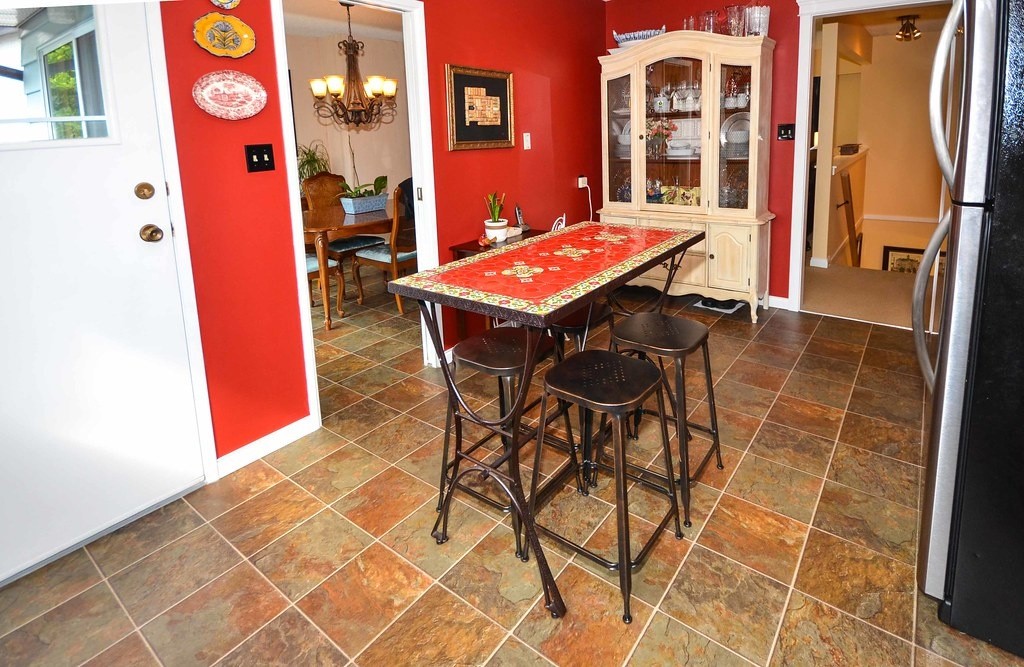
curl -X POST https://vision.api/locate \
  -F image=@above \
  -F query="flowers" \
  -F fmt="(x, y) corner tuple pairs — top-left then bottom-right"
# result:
(645, 114), (678, 141)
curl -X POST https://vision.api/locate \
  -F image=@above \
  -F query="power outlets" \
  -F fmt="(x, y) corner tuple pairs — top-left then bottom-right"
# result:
(578, 177), (587, 189)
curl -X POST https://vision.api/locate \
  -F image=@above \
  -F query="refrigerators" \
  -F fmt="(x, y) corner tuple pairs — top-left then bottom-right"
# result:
(910, 0), (1024, 662)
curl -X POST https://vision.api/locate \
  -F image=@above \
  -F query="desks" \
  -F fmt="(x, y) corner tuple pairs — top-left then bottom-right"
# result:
(387, 220), (704, 620)
(303, 199), (395, 331)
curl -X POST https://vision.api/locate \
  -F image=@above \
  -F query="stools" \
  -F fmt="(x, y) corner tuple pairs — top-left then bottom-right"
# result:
(435, 304), (725, 625)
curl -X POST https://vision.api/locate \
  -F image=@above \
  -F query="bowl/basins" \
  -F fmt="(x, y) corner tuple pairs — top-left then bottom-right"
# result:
(617, 135), (631, 145)
(666, 149), (696, 155)
(725, 131), (749, 144)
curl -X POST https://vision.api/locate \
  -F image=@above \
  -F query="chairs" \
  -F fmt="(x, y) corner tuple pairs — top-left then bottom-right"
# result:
(302, 171), (418, 319)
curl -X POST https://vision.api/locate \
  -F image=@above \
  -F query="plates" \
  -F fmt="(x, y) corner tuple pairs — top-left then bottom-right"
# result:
(191, 69), (268, 120)
(719, 112), (749, 148)
(613, 111), (630, 115)
(622, 121), (630, 134)
(623, 78), (654, 111)
(210, 0), (242, 9)
(192, 11), (255, 59)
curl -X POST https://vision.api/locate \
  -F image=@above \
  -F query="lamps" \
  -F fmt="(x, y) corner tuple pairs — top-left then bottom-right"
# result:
(896, 14), (922, 40)
(308, 2), (398, 127)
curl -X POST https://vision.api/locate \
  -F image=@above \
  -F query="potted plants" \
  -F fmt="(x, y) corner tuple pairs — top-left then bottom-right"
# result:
(330, 175), (389, 215)
(484, 191), (508, 243)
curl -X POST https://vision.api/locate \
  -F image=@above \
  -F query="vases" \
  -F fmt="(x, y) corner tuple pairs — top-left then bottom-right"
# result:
(646, 138), (666, 160)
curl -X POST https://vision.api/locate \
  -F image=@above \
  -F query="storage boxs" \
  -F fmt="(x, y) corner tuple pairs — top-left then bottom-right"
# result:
(661, 186), (700, 205)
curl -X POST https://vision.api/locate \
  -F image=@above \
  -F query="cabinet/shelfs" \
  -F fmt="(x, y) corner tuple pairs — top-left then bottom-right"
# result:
(448, 229), (551, 339)
(595, 31), (776, 323)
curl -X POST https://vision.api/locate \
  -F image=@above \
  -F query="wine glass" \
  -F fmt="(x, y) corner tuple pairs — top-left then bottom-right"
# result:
(662, 79), (704, 114)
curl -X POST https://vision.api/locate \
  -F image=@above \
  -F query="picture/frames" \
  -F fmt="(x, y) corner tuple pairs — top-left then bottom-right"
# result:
(882, 246), (946, 277)
(445, 63), (514, 152)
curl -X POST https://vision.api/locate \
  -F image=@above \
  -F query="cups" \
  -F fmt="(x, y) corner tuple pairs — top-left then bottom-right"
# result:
(737, 93), (749, 108)
(725, 97), (737, 109)
(684, 5), (770, 37)
(654, 97), (671, 113)
